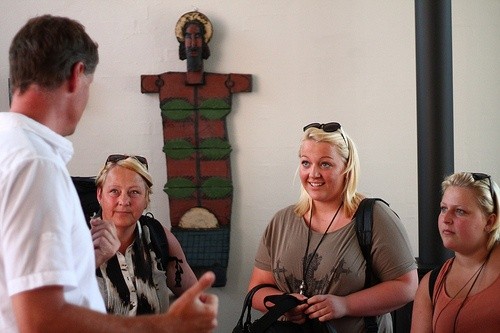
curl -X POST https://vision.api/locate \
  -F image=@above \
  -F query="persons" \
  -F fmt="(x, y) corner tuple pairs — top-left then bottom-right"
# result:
(412, 171), (500, 332)
(89, 154), (199, 318)
(0, 14), (219, 333)
(140, 8), (253, 289)
(246, 123), (418, 333)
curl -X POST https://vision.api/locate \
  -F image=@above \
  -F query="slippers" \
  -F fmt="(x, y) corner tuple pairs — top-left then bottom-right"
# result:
(303, 123), (345, 142)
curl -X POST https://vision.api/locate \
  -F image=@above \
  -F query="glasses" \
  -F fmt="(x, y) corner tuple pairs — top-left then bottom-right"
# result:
(105, 155), (148, 170)
(472, 173), (493, 198)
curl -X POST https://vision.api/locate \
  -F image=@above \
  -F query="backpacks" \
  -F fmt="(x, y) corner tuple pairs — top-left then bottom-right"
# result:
(233, 283), (336, 333)
